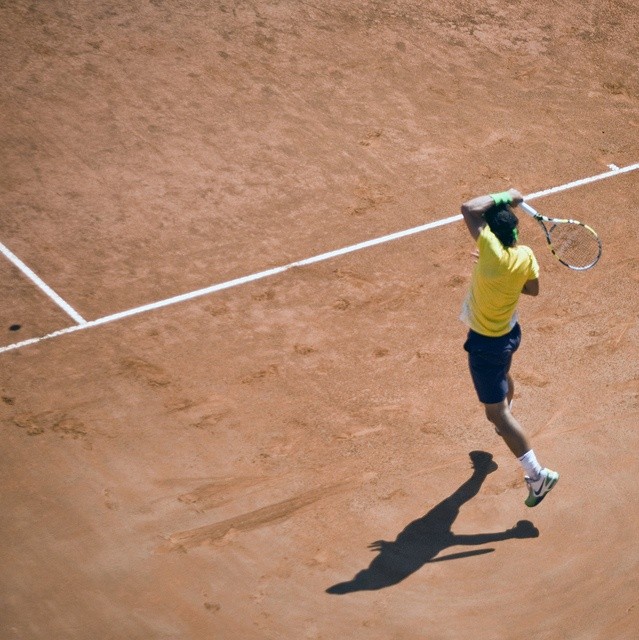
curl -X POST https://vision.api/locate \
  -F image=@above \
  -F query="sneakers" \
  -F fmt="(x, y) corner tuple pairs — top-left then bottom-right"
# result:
(524, 467), (560, 507)
(495, 399), (512, 435)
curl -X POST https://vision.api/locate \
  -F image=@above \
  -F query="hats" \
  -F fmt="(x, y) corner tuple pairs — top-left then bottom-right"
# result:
(485, 204), (518, 246)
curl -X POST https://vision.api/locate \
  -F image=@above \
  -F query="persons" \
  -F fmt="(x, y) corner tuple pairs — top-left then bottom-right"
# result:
(458, 188), (560, 508)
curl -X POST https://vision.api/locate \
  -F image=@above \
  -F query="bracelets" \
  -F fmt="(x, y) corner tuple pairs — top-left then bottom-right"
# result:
(489, 191), (513, 206)
